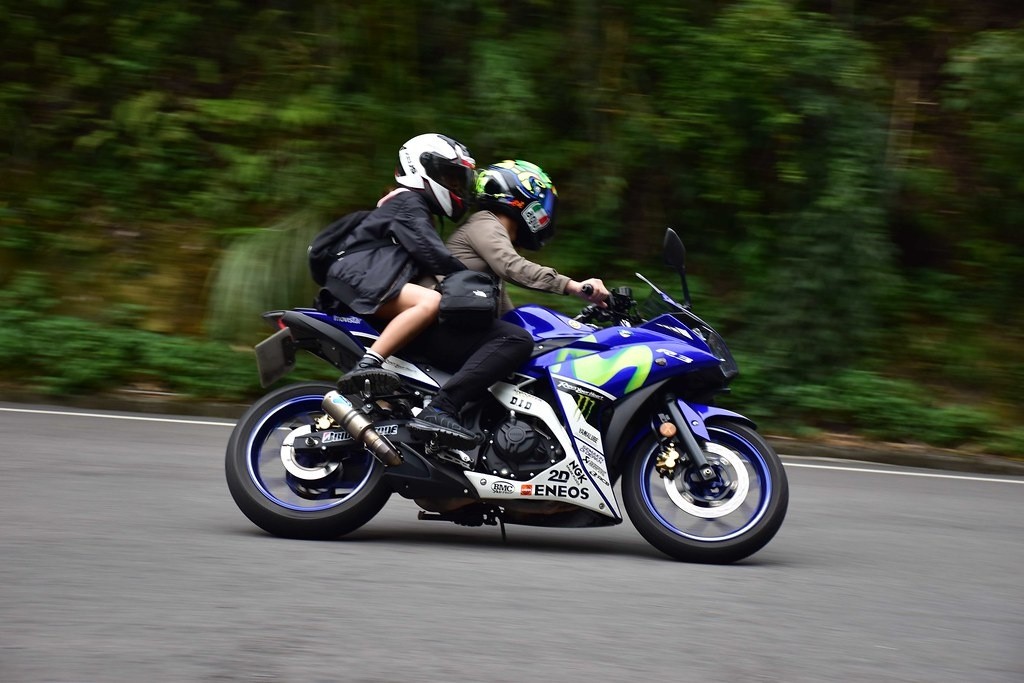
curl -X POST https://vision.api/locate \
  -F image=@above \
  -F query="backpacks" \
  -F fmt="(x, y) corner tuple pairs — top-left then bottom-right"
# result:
(306, 186), (430, 288)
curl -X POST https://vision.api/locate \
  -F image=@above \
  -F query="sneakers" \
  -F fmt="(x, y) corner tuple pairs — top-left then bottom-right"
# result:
(405, 405), (478, 447)
(338, 357), (400, 396)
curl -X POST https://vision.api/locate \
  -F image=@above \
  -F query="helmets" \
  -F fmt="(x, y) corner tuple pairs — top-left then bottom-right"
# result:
(393, 133), (478, 224)
(472, 160), (560, 252)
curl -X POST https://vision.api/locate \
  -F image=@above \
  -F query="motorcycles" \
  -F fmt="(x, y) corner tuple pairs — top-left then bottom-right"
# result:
(223, 280), (790, 567)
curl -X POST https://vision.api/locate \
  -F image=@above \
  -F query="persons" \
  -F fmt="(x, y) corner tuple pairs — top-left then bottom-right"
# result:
(324, 133), (612, 446)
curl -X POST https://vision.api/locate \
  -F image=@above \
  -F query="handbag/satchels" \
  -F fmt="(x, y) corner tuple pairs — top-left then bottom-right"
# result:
(438, 272), (499, 314)
